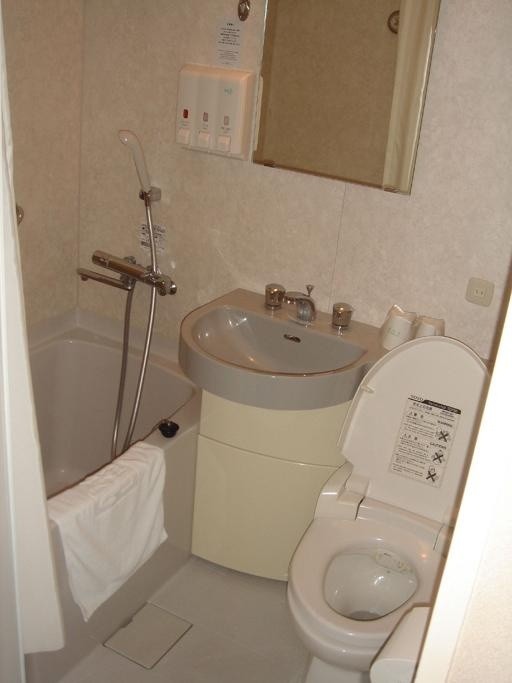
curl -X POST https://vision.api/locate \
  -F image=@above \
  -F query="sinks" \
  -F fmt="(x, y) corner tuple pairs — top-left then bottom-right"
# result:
(179, 287), (370, 411)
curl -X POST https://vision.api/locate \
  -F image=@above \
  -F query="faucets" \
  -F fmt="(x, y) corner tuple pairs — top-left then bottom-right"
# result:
(281, 290), (318, 322)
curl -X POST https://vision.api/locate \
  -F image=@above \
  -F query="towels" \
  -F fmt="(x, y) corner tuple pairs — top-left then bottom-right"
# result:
(45, 440), (168, 623)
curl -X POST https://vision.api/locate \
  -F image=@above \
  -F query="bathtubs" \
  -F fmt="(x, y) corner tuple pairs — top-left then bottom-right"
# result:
(25, 307), (201, 683)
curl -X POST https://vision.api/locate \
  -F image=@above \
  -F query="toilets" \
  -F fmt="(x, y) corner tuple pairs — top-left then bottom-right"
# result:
(281, 335), (491, 683)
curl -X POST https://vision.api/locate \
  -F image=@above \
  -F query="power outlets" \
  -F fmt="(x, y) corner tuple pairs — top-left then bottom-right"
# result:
(465, 277), (495, 307)
